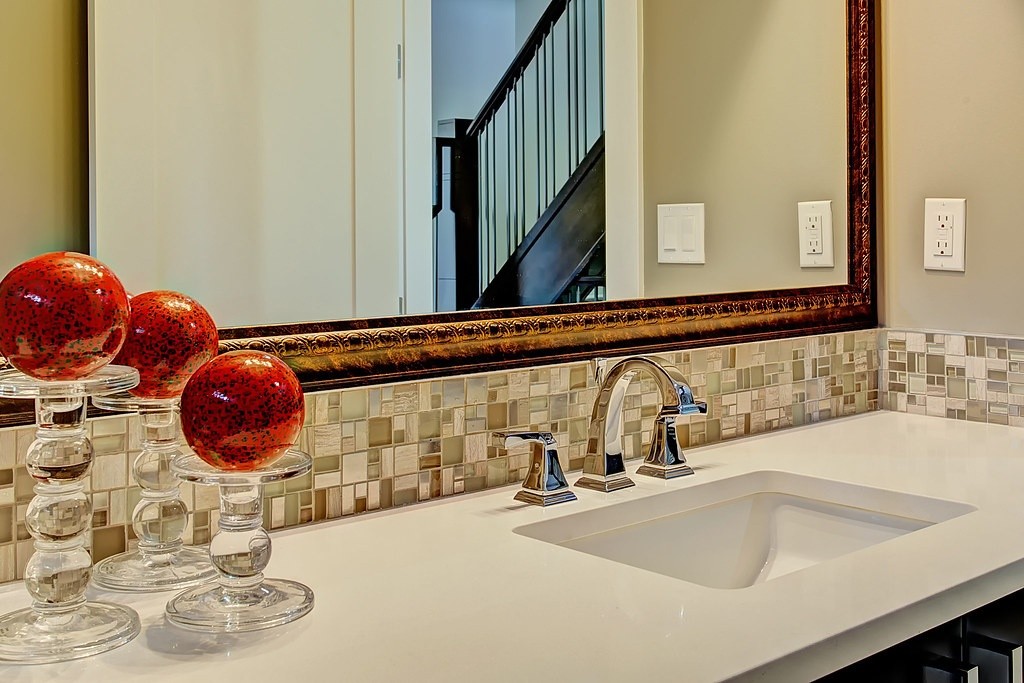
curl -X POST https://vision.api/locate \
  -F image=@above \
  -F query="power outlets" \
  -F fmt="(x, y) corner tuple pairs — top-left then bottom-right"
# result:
(924, 198), (965, 272)
(798, 199), (835, 268)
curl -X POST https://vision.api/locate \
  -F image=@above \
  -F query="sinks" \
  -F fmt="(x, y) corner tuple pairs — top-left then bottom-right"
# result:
(509, 468), (979, 591)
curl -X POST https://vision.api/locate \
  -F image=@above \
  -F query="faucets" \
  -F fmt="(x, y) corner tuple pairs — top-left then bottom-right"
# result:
(572, 355), (708, 493)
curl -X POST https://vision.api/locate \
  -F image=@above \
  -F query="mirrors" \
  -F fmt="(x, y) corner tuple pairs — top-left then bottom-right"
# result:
(0, 0), (878, 427)
